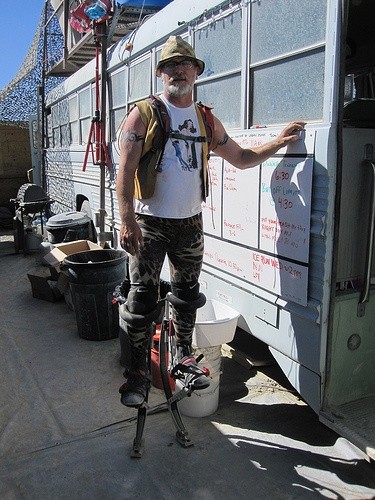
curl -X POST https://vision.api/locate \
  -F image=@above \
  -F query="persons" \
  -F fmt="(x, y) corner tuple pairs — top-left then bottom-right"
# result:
(116, 35), (306, 408)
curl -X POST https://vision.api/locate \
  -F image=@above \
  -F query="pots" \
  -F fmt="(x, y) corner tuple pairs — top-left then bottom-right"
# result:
(45, 211), (93, 244)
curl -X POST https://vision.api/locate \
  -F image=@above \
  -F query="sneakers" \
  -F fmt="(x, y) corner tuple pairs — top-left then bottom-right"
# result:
(124, 372), (146, 406)
(173, 356), (210, 389)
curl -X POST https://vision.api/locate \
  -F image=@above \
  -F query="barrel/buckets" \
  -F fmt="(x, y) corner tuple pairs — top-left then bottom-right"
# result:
(149, 324), (175, 391)
(172, 344), (223, 418)
(60, 249), (127, 341)
(26, 233), (43, 250)
(114, 276), (173, 369)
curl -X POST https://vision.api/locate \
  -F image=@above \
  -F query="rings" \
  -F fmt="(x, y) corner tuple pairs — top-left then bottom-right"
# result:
(121, 241), (126, 245)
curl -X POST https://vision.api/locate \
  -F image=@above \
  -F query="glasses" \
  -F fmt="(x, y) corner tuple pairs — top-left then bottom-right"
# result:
(164, 60), (192, 70)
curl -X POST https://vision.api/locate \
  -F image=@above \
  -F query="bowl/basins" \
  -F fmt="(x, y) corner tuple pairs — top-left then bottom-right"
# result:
(191, 298), (241, 347)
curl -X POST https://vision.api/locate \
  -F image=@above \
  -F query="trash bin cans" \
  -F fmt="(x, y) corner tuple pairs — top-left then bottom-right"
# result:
(60, 249), (128, 340)
(113, 282), (168, 325)
(46, 211), (95, 254)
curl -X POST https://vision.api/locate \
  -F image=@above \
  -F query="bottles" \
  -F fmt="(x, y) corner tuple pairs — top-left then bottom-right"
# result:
(31, 216), (48, 241)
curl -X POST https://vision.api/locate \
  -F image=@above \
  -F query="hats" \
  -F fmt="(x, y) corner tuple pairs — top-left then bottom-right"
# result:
(156, 35), (205, 77)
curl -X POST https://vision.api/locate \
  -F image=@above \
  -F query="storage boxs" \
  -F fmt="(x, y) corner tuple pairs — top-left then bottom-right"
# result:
(26, 239), (103, 303)
(192, 300), (241, 347)
